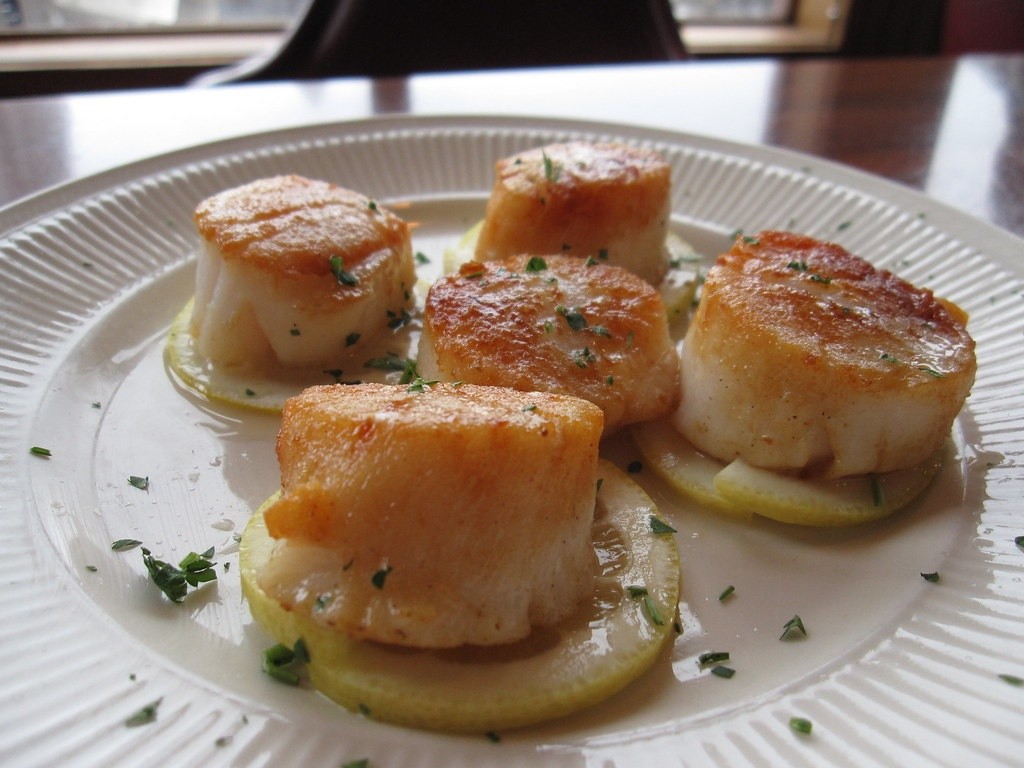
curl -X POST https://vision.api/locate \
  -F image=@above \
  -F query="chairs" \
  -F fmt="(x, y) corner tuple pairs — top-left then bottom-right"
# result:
(186, 0), (690, 92)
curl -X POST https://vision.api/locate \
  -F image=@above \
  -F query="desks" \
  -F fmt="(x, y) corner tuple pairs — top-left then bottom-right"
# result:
(0, 51), (1024, 243)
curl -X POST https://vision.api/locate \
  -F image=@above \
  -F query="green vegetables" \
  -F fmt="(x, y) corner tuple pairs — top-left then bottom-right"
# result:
(144, 545), (217, 603)
(261, 638), (310, 684)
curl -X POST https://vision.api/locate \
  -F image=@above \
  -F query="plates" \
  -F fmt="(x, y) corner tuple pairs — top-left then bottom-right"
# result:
(0, 113), (1024, 768)
